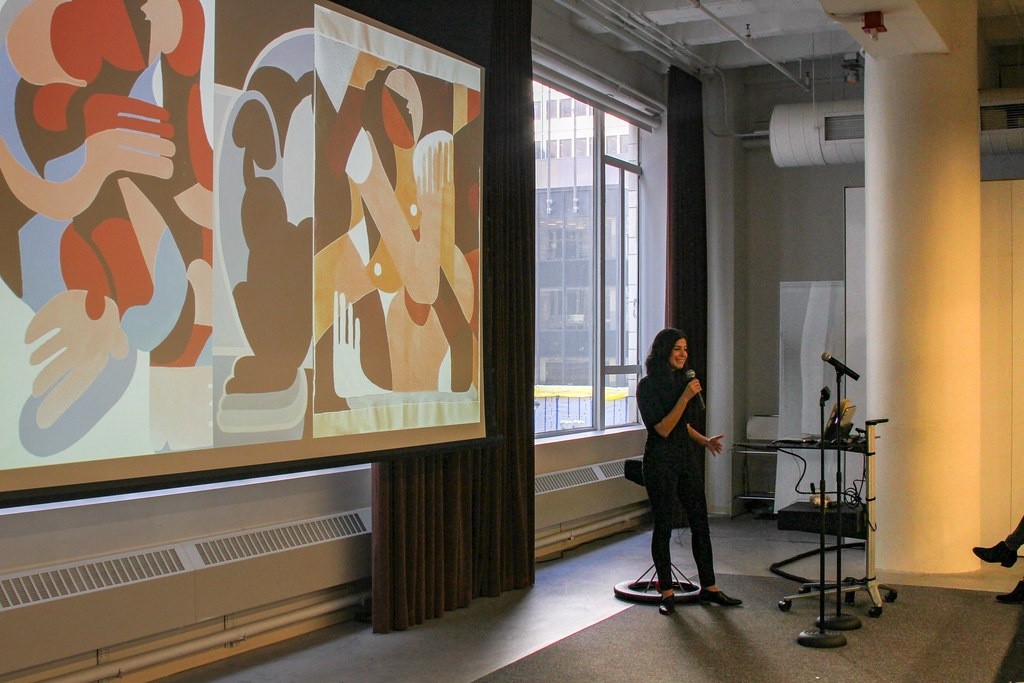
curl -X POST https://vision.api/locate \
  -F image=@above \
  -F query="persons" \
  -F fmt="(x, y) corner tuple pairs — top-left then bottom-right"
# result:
(634, 327), (743, 615)
(972, 514), (1024, 604)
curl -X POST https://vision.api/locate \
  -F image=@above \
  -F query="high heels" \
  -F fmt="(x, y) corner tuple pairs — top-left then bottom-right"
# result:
(659, 592), (676, 615)
(698, 587), (742, 606)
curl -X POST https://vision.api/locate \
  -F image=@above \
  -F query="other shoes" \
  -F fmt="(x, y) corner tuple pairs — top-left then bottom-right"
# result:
(972, 541), (1018, 568)
(996, 579), (1024, 605)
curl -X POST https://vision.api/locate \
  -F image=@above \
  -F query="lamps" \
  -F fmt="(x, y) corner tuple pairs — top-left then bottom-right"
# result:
(840, 53), (864, 83)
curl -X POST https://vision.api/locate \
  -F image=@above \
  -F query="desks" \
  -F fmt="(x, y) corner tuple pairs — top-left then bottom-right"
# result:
(735, 439), (777, 516)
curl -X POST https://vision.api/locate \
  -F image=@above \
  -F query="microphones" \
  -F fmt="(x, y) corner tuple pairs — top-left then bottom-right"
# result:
(685, 369), (705, 411)
(821, 352), (860, 381)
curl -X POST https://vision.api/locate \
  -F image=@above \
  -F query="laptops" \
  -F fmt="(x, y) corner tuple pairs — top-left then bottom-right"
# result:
(777, 399), (851, 443)
